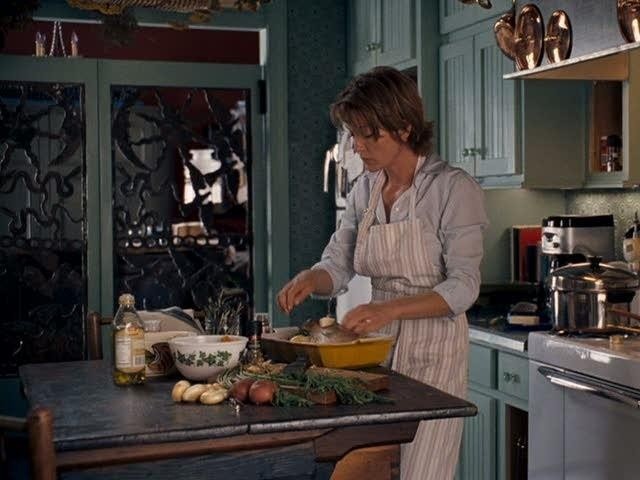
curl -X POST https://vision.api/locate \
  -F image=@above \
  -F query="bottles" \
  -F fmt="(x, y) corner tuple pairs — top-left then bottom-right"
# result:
(254, 312), (273, 335)
(606, 134), (623, 173)
(111, 293), (147, 387)
(247, 321), (262, 364)
(599, 136), (607, 174)
(141, 318), (161, 332)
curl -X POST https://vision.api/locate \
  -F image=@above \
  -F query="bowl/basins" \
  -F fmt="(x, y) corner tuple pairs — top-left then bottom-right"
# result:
(259, 322), (396, 369)
(167, 333), (250, 382)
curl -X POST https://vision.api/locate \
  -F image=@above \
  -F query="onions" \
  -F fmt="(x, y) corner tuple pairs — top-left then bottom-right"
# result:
(229, 379), (276, 404)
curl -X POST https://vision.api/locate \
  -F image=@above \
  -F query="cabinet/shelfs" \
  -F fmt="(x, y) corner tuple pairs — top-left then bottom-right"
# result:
(497, 345), (530, 480)
(435, 0), (587, 197)
(334, 0), (437, 131)
(577, 77), (640, 191)
(455, 340), (499, 479)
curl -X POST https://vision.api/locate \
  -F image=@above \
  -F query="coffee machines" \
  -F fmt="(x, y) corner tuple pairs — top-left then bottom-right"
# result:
(538, 211), (616, 325)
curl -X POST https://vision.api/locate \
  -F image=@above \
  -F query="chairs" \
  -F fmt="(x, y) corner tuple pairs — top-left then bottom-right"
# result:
(83, 307), (117, 365)
(1, 403), (60, 480)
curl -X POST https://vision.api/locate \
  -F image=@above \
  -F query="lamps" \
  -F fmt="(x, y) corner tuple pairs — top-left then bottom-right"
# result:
(33, 20), (82, 96)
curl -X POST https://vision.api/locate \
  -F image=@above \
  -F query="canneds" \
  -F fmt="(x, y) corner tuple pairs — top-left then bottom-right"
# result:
(600, 135), (608, 172)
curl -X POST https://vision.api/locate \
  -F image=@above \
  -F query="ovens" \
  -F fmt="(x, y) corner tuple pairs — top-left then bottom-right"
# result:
(528, 334), (640, 480)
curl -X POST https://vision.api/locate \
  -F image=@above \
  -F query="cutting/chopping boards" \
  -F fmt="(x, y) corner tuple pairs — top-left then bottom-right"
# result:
(237, 356), (390, 406)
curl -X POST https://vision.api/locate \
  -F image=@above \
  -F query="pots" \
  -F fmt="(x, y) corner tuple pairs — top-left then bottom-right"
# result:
(541, 250), (639, 337)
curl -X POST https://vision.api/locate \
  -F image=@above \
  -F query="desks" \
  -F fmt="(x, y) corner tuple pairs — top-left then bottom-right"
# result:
(12, 345), (480, 480)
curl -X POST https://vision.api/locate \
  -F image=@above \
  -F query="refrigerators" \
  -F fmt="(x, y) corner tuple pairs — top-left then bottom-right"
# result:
(323, 113), (371, 327)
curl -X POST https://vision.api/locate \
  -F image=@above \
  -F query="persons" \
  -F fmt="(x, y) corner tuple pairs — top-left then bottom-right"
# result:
(274, 65), (486, 480)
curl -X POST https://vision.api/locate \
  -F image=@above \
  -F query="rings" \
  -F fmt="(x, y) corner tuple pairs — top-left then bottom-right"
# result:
(366, 319), (372, 325)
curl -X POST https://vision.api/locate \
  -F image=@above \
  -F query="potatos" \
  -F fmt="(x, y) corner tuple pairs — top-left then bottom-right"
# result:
(320, 317), (335, 327)
(171, 379), (228, 405)
(290, 335), (310, 343)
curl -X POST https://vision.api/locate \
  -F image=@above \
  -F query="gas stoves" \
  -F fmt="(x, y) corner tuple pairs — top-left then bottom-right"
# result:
(543, 323), (640, 360)
